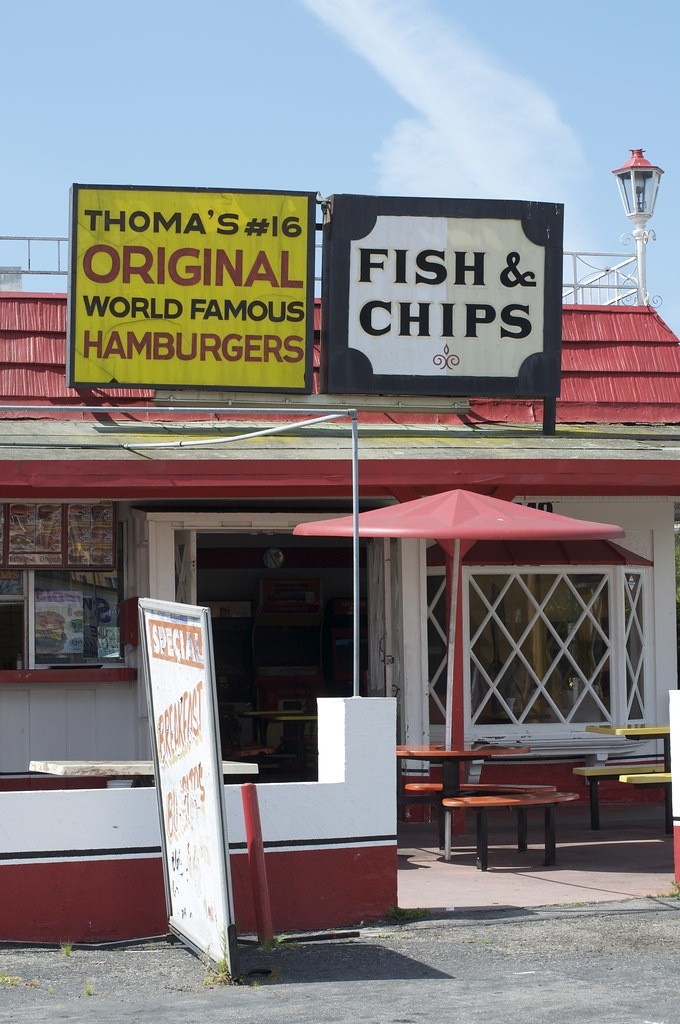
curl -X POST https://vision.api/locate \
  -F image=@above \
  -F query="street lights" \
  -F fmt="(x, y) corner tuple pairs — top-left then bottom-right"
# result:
(611, 147), (664, 306)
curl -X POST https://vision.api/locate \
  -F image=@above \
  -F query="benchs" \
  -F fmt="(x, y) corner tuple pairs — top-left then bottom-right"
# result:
(571, 765), (666, 835)
(442, 792), (580, 870)
(618, 773), (672, 787)
(404, 785), (557, 854)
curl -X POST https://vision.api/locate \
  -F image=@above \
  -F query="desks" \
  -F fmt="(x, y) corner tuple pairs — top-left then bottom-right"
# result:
(393, 745), (533, 861)
(585, 726), (673, 835)
(25, 759), (261, 788)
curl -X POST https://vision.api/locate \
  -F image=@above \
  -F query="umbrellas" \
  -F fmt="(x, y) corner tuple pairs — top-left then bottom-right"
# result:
(292, 490), (625, 860)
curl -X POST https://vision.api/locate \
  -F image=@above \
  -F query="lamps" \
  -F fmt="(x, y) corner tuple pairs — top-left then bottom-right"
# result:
(612, 147), (665, 224)
